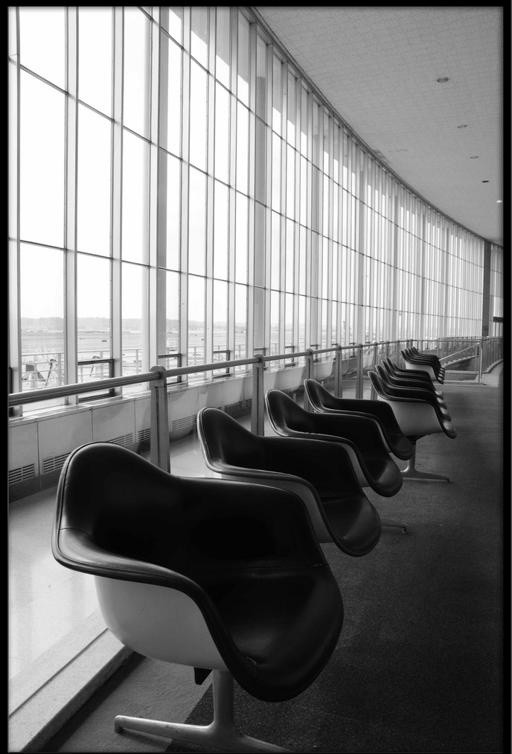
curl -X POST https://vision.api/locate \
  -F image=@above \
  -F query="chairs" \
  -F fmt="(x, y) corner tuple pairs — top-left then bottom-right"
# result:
(268, 390), (404, 498)
(368, 347), (456, 488)
(52, 440), (344, 754)
(196, 407), (382, 557)
(303, 378), (416, 463)
